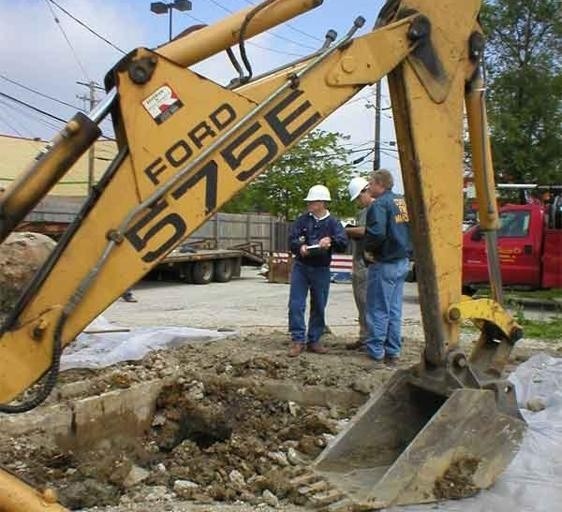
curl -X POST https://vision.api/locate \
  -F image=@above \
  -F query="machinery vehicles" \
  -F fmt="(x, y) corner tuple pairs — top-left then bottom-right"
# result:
(0, 0), (529, 512)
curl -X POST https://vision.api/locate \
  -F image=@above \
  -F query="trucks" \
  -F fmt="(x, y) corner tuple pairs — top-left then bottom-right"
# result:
(158, 248), (241, 284)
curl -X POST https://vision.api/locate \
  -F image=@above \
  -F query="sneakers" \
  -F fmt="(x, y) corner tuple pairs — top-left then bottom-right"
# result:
(306, 342), (330, 355)
(345, 341), (363, 350)
(287, 343), (304, 358)
(363, 352), (399, 369)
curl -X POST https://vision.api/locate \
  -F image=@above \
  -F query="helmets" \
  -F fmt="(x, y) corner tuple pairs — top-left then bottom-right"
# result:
(303, 185), (333, 203)
(347, 177), (369, 202)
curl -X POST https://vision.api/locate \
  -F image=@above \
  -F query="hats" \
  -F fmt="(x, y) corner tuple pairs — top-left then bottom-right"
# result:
(369, 169), (394, 190)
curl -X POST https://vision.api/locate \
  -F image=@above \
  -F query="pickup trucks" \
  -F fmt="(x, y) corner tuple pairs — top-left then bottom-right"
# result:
(463, 202), (562, 298)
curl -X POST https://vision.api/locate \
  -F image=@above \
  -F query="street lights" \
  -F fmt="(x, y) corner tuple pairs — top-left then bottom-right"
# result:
(151, 0), (192, 43)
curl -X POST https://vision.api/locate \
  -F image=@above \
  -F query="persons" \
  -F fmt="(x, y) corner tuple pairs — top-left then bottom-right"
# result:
(341, 177), (372, 350)
(280, 184), (349, 356)
(358, 169), (413, 362)
